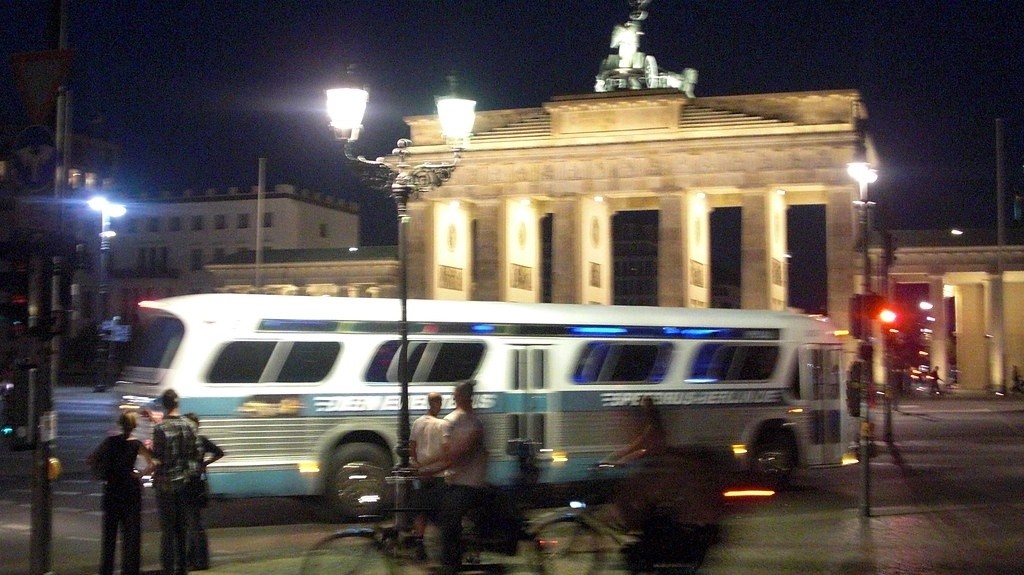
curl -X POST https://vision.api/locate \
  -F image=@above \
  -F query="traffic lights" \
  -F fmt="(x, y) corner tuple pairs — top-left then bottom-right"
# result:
(843, 361), (860, 417)
(879, 303), (899, 328)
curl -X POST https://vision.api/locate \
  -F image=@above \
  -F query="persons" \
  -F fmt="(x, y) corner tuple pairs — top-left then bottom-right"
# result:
(1012, 364), (1024, 394)
(929, 366), (941, 394)
(85, 412), (161, 575)
(407, 392), (449, 562)
(143, 389), (223, 575)
(605, 395), (665, 465)
(413, 380), (486, 575)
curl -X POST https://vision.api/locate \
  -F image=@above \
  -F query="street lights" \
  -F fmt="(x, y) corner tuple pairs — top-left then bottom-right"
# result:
(324, 59), (477, 534)
(847, 161), (878, 458)
(87, 198), (129, 394)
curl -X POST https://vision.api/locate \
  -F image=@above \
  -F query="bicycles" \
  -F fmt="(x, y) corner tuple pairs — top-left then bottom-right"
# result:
(526, 461), (698, 575)
(298, 448), (478, 575)
(929, 384), (953, 403)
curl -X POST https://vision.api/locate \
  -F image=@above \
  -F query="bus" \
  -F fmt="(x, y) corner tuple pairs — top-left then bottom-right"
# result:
(117, 294), (874, 506)
